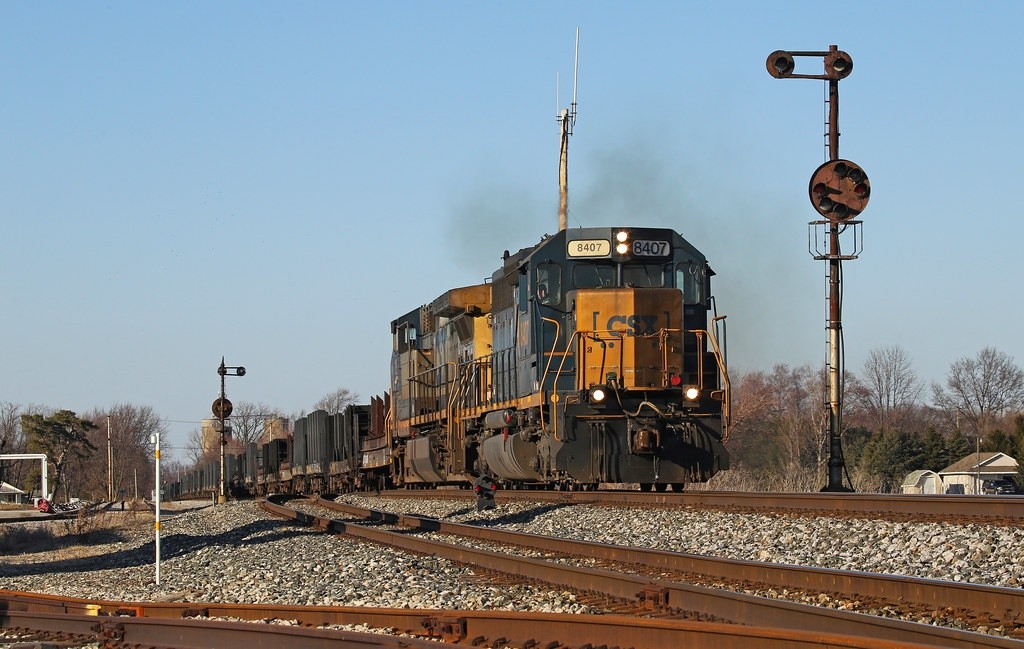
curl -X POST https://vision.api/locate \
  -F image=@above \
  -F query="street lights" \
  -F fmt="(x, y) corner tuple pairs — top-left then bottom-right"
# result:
(977, 437), (982, 495)
(151, 432), (160, 586)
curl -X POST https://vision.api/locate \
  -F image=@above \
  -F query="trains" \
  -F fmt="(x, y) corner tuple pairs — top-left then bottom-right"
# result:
(164, 26), (731, 491)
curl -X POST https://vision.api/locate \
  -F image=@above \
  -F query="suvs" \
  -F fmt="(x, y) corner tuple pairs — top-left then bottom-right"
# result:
(982, 480), (1015, 495)
